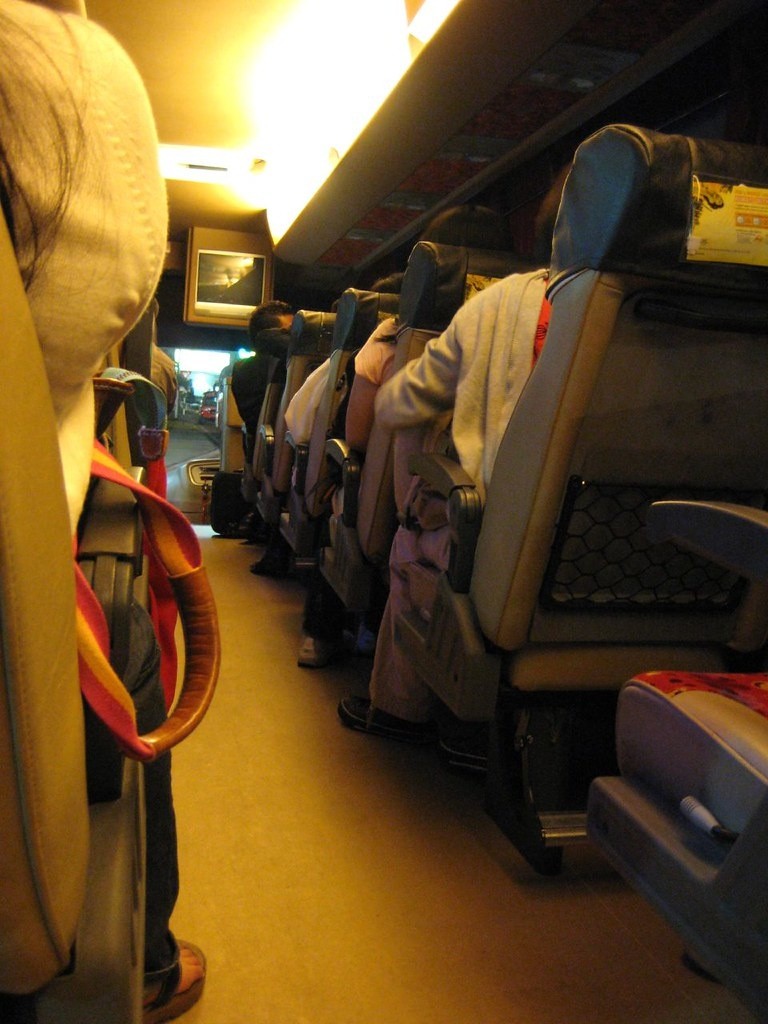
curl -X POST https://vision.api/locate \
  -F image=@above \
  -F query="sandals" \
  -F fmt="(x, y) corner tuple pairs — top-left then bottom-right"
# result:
(142, 939), (208, 1024)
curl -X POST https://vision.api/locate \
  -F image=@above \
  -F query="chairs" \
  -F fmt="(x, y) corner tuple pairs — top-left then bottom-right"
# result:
(222, 122), (768, 1024)
(0, 204), (148, 1024)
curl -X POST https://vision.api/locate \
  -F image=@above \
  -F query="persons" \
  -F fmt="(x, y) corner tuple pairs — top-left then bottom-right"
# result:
(0, 0), (210, 1024)
(335, 159), (574, 775)
(230, 199), (514, 665)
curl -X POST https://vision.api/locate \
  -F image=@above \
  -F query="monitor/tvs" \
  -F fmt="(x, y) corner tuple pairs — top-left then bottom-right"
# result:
(194, 249), (266, 320)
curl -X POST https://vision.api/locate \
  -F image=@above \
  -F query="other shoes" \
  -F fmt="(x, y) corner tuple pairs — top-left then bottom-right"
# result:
(296, 631), (336, 670)
(356, 613), (377, 657)
(338, 696), (425, 743)
(437, 737), (489, 777)
